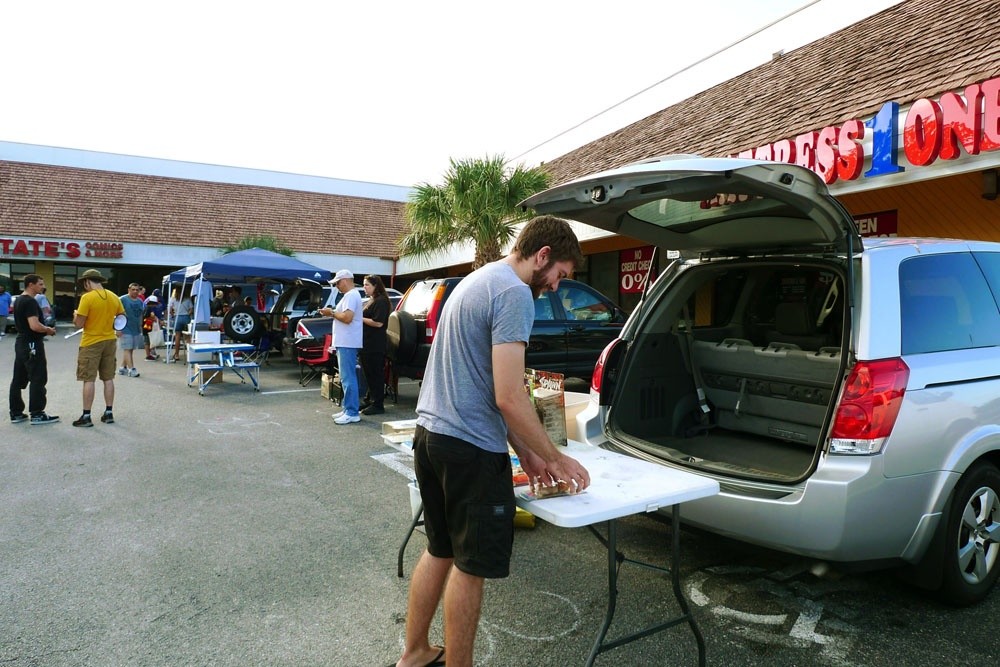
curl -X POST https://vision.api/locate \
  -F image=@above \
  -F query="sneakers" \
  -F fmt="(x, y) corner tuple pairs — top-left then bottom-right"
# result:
(73, 417), (93, 428)
(30, 414), (60, 425)
(101, 412), (114, 423)
(331, 397), (386, 424)
(11, 414), (28, 423)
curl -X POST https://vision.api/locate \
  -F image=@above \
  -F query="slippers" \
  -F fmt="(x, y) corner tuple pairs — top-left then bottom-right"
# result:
(386, 644), (444, 667)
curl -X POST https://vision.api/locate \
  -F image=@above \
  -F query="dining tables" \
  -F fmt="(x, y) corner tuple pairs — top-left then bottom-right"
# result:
(384, 429), (720, 667)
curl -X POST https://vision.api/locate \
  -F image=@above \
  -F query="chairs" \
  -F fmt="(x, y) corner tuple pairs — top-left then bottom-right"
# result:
(296, 334), (336, 387)
(243, 334), (271, 367)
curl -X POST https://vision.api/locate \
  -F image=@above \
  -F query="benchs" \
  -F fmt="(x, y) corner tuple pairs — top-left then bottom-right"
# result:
(196, 362), (261, 396)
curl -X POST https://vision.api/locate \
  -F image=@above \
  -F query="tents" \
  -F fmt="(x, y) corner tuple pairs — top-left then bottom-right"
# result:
(168, 248), (332, 361)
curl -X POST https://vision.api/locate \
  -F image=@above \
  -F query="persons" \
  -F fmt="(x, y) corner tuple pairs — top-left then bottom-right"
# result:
(386, 214), (592, 667)
(213, 290), (225, 317)
(244, 296), (253, 306)
(9, 273), (61, 425)
(0, 284), (12, 335)
(73, 269), (126, 427)
(168, 285), (193, 360)
(317, 269), (364, 425)
(142, 289), (167, 360)
(357, 275), (391, 415)
(118, 282), (146, 378)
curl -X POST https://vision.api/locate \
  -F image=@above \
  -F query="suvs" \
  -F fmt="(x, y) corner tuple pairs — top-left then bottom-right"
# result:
(383, 274), (635, 398)
(507, 153), (1000, 608)
(269, 284), (404, 361)
(205, 283), (279, 362)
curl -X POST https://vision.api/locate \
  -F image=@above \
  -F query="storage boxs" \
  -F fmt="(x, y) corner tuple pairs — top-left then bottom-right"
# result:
(407, 481), (427, 536)
(320, 378), (342, 399)
(562, 390), (591, 442)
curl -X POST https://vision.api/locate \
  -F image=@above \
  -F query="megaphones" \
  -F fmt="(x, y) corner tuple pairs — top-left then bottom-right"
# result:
(113, 313), (128, 331)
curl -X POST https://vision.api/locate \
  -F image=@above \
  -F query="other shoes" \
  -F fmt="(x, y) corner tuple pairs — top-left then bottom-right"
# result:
(119, 350), (179, 378)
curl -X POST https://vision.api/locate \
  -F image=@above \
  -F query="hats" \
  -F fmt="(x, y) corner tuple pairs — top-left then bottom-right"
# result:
(78, 268), (108, 281)
(328, 269), (354, 284)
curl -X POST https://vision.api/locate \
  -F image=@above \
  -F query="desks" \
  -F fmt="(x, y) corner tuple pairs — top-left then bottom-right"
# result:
(179, 330), (226, 366)
(189, 342), (255, 368)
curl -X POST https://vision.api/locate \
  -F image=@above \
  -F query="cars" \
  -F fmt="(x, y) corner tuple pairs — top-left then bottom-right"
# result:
(286, 295), (404, 377)
(6, 294), (55, 333)
